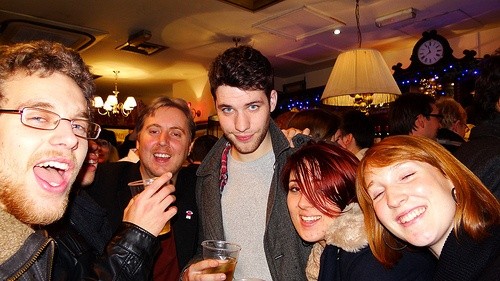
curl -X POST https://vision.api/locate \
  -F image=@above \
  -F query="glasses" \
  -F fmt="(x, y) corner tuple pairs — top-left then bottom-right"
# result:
(417, 113), (444, 123)
(0, 107), (102, 140)
(461, 122), (470, 133)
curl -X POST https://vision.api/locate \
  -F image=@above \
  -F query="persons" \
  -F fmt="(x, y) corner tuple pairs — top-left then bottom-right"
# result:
(280, 54), (500, 202)
(356, 136), (500, 281)
(95, 96), (199, 281)
(0, 41), (176, 281)
(283, 142), (391, 281)
(167, 135), (218, 269)
(181, 47), (314, 281)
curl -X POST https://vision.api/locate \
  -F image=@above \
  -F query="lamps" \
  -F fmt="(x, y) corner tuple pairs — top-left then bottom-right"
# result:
(375, 8), (418, 28)
(318, 0), (403, 116)
(91, 71), (137, 118)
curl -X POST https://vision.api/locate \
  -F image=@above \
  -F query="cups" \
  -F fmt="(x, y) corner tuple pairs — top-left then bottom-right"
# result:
(201, 240), (242, 281)
(128, 178), (170, 235)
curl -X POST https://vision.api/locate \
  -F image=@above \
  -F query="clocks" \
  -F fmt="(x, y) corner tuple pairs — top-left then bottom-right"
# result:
(415, 37), (445, 66)
